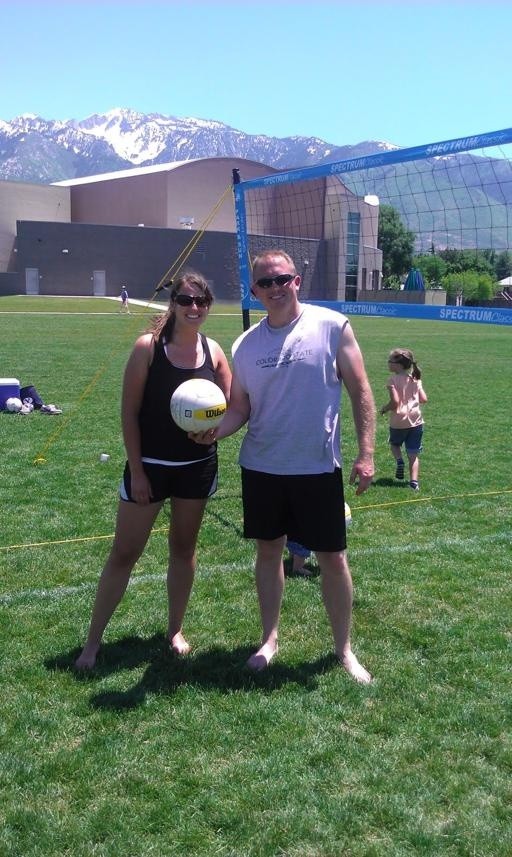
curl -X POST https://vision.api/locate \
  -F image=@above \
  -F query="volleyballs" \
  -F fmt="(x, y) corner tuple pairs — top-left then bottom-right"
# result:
(6, 398), (22, 414)
(171, 378), (226, 434)
(344, 500), (351, 527)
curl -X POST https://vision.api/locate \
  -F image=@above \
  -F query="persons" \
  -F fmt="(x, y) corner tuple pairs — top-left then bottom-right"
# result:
(382, 348), (428, 490)
(76, 273), (234, 668)
(186, 248), (378, 684)
(286, 538), (313, 577)
(120, 285), (130, 315)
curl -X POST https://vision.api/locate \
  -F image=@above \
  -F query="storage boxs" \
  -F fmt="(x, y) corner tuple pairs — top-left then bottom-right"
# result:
(0, 378), (21, 410)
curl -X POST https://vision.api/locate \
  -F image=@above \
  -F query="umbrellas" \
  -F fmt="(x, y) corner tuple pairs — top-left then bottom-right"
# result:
(403, 267), (424, 290)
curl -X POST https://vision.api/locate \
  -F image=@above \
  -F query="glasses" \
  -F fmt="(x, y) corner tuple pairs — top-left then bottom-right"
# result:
(253, 272), (296, 288)
(388, 359), (399, 364)
(174, 292), (211, 308)
